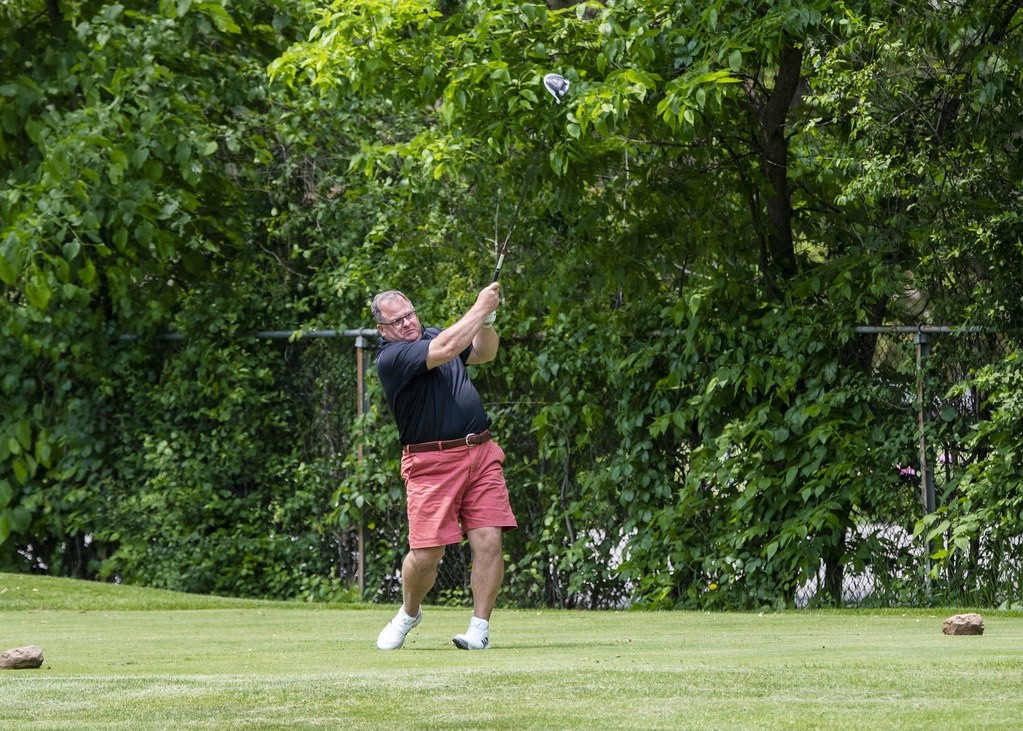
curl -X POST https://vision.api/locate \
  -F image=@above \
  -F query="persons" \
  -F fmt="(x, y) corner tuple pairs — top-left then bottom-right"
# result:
(371, 282), (518, 650)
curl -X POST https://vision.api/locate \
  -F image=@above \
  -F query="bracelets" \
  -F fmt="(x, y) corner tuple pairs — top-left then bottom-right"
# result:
(481, 311), (496, 329)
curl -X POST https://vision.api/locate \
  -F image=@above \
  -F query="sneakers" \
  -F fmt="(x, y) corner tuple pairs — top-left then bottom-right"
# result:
(376, 604), (423, 651)
(452, 629), (490, 649)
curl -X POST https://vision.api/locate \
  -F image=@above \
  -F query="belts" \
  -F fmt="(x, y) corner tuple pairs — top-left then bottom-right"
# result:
(403, 429), (492, 453)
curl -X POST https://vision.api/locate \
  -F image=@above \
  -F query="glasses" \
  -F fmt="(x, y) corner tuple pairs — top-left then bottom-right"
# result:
(376, 309), (417, 329)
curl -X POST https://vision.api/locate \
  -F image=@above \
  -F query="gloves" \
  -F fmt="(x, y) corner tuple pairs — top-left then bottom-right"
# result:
(481, 311), (496, 328)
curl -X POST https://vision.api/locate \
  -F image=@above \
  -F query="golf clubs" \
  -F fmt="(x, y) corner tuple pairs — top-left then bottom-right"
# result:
(490, 73), (571, 287)
